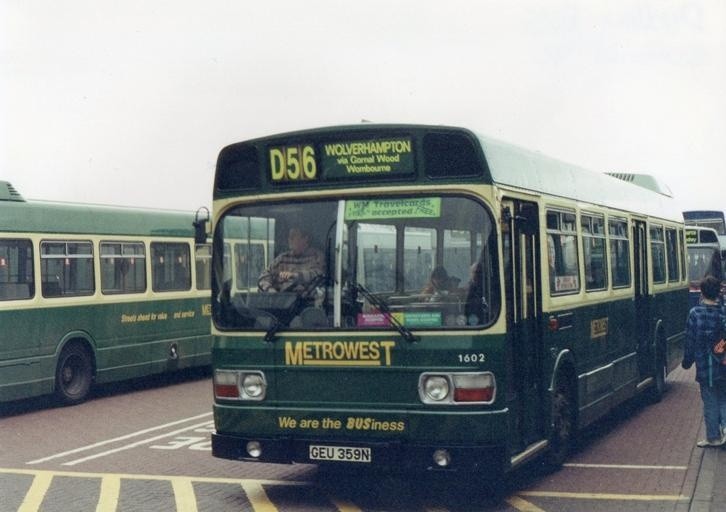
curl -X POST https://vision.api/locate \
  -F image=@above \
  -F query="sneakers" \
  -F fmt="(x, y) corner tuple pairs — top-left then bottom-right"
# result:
(697, 427), (726, 447)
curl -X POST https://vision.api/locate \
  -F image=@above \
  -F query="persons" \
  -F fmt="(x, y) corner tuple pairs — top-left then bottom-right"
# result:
(420, 265), (453, 302)
(258, 225), (327, 293)
(682, 276), (726, 447)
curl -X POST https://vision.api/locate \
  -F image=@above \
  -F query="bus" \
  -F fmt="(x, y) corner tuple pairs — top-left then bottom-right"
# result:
(686, 226), (720, 307)
(210, 124), (687, 506)
(1, 181), (274, 405)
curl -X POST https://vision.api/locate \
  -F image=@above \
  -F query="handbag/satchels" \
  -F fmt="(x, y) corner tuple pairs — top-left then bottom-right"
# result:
(711, 331), (726, 365)
